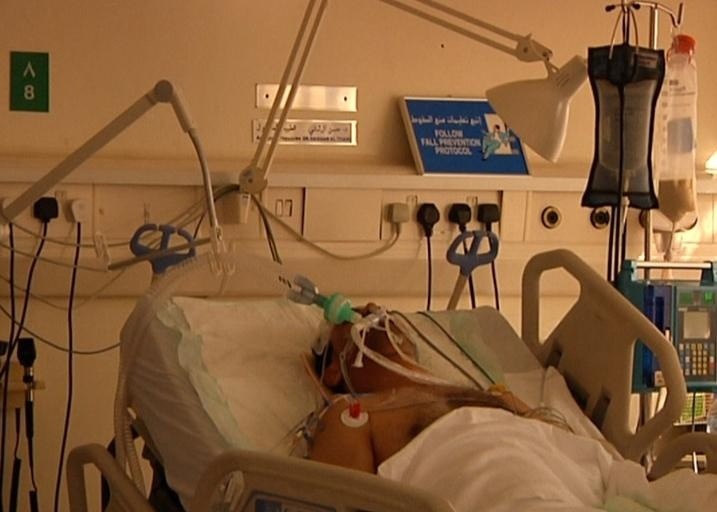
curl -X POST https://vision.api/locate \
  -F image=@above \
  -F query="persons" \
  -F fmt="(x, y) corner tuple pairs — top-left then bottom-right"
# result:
(478, 124), (511, 159)
(306, 302), (569, 477)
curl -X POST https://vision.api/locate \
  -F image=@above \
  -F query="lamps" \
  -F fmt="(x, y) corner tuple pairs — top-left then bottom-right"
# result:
(210, 0), (588, 227)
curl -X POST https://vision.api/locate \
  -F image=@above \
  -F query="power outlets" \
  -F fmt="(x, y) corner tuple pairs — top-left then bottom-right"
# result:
(381, 190), (420, 241)
(0, 181), (30, 239)
(30, 181), (95, 239)
(416, 191), (501, 243)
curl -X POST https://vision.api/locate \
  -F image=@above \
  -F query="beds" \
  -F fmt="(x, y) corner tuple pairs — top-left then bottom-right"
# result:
(66, 249), (717, 512)
